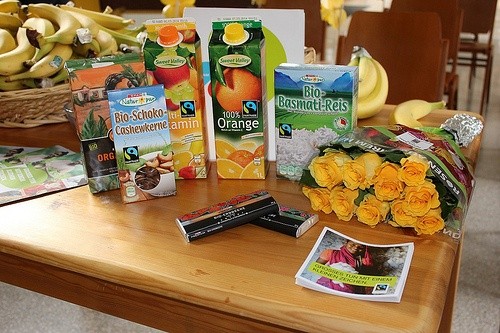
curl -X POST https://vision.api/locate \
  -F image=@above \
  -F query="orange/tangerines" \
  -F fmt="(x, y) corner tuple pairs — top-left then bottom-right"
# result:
(172, 140), (204, 171)
(192, 46), (205, 109)
(216, 68), (262, 112)
(215, 140), (264, 179)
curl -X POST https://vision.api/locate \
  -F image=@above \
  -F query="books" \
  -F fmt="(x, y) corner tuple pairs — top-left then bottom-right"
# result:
(295, 227), (416, 302)
(0, 144), (88, 206)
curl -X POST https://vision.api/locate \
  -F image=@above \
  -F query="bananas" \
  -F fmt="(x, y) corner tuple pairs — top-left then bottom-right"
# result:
(389, 99), (445, 128)
(347, 46), (388, 118)
(0, 0), (146, 91)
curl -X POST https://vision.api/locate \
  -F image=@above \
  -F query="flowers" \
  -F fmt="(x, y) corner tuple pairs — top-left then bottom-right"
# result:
(302, 151), (447, 236)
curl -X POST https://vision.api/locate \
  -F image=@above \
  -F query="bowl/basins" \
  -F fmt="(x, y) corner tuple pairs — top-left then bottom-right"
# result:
(64, 101), (76, 132)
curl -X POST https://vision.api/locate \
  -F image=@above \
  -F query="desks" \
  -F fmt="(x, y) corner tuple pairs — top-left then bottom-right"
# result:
(0, 103), (484, 333)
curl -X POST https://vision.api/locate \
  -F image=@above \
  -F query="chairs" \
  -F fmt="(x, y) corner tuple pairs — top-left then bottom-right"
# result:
(337, 0), (497, 115)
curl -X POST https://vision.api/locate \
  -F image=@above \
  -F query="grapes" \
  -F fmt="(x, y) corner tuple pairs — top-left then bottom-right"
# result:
(164, 80), (195, 105)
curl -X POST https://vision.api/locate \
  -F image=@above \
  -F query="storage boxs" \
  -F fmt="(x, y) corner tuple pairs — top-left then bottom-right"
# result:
(274, 63), (359, 181)
(108, 84), (177, 205)
(65, 54), (145, 193)
(176, 190), (278, 241)
(254, 208), (318, 238)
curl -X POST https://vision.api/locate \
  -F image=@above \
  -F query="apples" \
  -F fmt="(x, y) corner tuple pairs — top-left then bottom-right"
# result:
(179, 166), (196, 179)
(146, 56), (189, 111)
